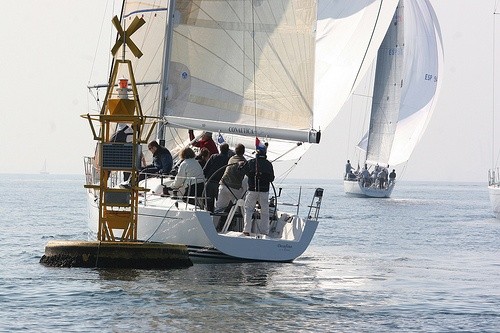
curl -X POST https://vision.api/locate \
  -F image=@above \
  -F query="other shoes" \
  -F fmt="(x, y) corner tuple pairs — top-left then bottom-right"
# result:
(259, 235), (267, 239)
(239, 232), (249, 237)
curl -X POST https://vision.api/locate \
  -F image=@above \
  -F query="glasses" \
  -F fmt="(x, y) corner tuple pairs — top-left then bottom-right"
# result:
(149, 146), (154, 150)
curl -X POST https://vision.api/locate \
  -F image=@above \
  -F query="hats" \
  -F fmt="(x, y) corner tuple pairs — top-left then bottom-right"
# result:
(256, 144), (266, 153)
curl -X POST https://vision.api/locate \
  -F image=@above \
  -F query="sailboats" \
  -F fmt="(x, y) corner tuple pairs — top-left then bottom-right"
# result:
(343, 1), (446, 198)
(79, 0), (323, 263)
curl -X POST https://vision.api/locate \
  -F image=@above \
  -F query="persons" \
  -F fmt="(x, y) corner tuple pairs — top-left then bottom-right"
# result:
(344, 159), (396, 189)
(212, 144), (247, 228)
(188, 129), (219, 155)
(204, 143), (232, 213)
(170, 148), (204, 205)
(237, 141), (274, 237)
(196, 148), (210, 167)
(119, 140), (173, 189)
